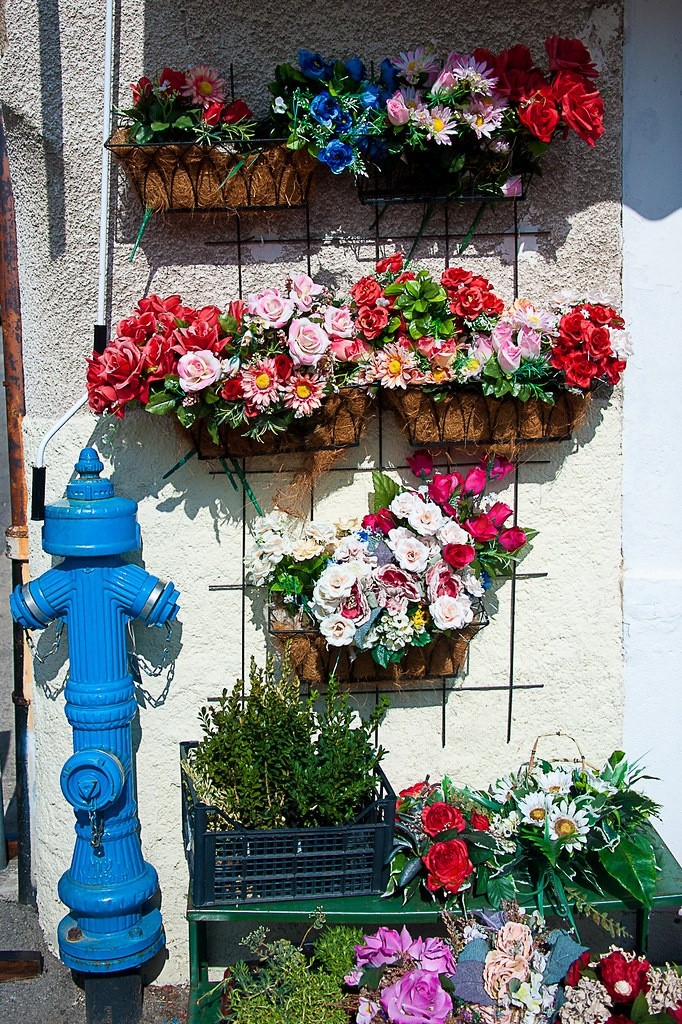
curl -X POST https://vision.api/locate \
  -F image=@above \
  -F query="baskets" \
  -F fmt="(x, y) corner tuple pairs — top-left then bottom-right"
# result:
(179, 740), (395, 909)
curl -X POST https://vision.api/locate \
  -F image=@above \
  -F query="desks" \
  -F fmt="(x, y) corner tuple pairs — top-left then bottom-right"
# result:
(185, 815), (682, 1024)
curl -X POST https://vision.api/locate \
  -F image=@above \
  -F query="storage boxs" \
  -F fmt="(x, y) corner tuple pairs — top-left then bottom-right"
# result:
(180, 741), (396, 909)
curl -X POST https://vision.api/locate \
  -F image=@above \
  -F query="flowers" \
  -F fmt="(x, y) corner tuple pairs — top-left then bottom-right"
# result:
(86, 251), (627, 446)
(107, 61), (258, 149)
(385, 735), (661, 941)
(244, 449), (542, 666)
(267, 35), (605, 194)
(344, 903), (682, 1024)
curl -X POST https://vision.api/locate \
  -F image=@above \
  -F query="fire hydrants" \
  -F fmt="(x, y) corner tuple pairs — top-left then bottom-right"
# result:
(7, 446), (181, 974)
